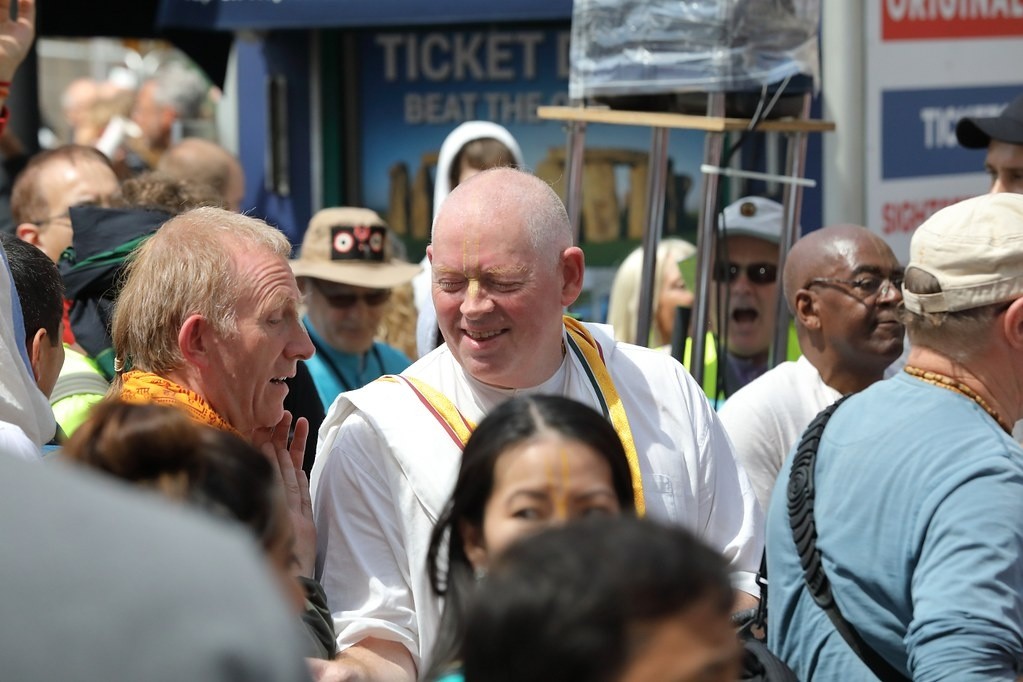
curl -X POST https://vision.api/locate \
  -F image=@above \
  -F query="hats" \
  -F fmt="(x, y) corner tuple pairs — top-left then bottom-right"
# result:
(955, 92), (1023, 151)
(287, 204), (426, 291)
(900, 190), (1023, 318)
(718, 194), (803, 251)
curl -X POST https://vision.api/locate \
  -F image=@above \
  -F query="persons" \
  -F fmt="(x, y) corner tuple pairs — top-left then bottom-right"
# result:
(0, 0), (526, 682)
(463, 518), (742, 682)
(761, 193), (1023, 682)
(419, 395), (638, 682)
(305, 167), (762, 682)
(0, 251), (326, 682)
(956, 97), (1023, 195)
(607, 196), (905, 639)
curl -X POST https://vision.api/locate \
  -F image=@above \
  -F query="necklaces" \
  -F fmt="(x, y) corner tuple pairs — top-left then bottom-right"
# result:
(902, 365), (1014, 439)
(346, 351), (368, 387)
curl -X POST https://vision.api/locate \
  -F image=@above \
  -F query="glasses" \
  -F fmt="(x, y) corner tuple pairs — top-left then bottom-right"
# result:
(26, 191), (127, 226)
(800, 273), (906, 295)
(308, 277), (394, 310)
(712, 260), (778, 285)
